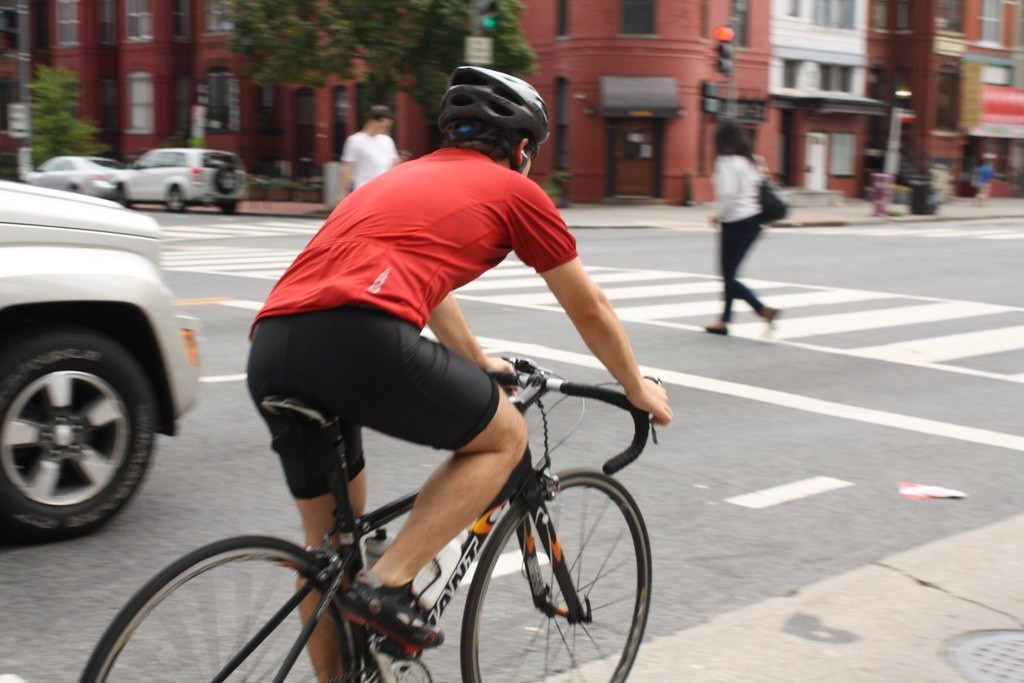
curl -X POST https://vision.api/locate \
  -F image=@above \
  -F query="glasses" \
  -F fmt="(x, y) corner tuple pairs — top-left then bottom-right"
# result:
(528, 140), (540, 161)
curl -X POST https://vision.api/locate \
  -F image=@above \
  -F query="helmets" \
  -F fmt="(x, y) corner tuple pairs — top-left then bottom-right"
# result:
(435, 64), (552, 144)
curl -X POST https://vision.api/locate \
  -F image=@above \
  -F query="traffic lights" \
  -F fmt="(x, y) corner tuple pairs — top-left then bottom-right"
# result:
(714, 23), (737, 77)
(699, 80), (721, 115)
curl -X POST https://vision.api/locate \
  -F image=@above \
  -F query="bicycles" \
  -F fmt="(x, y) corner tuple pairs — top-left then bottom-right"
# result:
(69, 349), (670, 683)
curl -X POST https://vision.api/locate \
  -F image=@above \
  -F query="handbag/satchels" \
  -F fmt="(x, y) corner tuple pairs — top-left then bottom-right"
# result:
(756, 178), (792, 225)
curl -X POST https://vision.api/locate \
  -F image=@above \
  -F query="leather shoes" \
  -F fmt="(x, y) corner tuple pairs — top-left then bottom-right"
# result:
(706, 324), (730, 336)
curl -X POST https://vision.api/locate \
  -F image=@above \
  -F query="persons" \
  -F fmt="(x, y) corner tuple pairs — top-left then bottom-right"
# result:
(246, 64), (674, 683)
(704, 119), (779, 336)
(971, 155), (996, 207)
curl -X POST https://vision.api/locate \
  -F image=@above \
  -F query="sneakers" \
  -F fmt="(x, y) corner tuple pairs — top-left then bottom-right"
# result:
(339, 571), (445, 651)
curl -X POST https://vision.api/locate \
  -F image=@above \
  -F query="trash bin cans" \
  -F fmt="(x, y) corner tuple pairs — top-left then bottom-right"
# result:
(908, 178), (937, 215)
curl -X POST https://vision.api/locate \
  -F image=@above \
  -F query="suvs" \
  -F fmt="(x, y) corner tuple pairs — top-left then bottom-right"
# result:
(112, 148), (249, 212)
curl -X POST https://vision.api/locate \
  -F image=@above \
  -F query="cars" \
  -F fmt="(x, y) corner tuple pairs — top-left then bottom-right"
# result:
(26, 156), (134, 201)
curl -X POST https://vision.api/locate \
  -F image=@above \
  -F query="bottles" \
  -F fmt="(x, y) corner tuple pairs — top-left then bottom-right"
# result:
(364, 527), (398, 572)
(410, 527), (467, 611)
